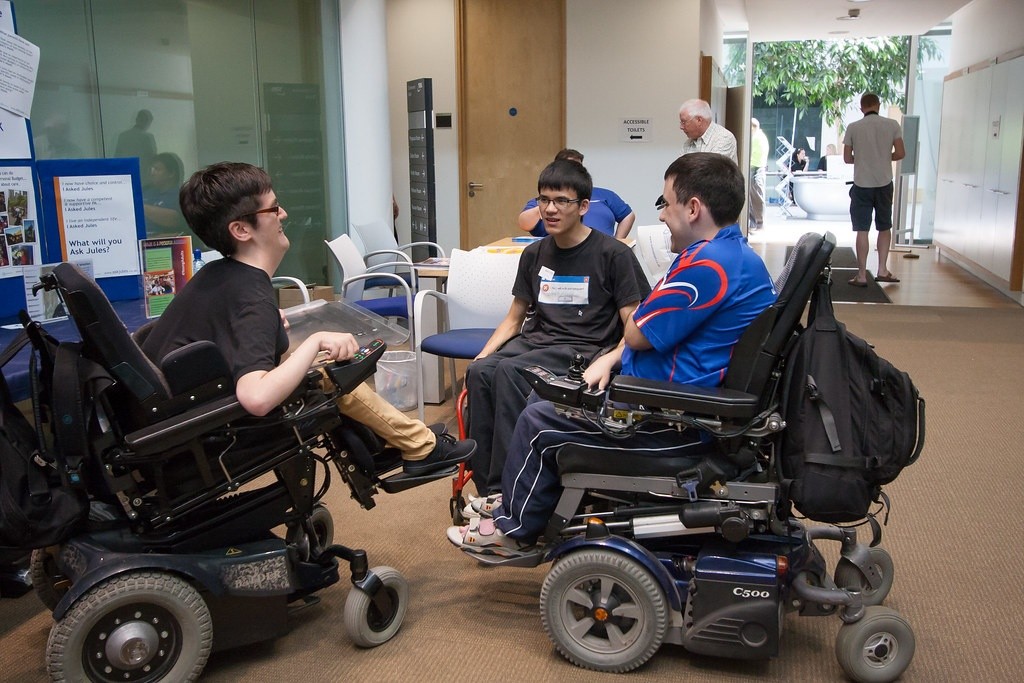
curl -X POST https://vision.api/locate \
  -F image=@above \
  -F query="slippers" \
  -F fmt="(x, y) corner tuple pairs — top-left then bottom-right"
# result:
(875, 271), (900, 283)
(848, 277), (867, 286)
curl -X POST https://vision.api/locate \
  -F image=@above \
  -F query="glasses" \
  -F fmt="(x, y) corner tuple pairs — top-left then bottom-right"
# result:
(534, 195), (581, 209)
(227, 202), (280, 238)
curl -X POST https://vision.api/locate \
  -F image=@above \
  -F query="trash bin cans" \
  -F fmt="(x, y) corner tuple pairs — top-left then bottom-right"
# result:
(374, 351), (417, 412)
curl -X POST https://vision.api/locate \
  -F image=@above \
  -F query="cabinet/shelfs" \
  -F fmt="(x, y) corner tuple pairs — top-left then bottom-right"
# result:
(932, 47), (1024, 292)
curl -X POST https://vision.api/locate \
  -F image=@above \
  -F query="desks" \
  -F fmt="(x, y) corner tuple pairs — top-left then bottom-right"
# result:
(416, 236), (636, 405)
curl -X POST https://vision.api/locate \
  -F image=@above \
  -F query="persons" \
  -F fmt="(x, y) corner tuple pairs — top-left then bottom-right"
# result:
(783, 148), (809, 206)
(133, 161), (478, 475)
(112, 110), (157, 160)
(516, 148), (635, 242)
(462, 162), (652, 518)
(679, 98), (739, 167)
(447, 152), (777, 558)
(43, 115), (84, 159)
(817, 144), (837, 172)
(150, 276), (173, 294)
(843, 93), (906, 287)
(141, 152), (185, 231)
(748, 118), (770, 230)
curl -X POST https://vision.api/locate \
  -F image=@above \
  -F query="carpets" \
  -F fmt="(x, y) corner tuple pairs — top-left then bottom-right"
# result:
(784, 246), (895, 303)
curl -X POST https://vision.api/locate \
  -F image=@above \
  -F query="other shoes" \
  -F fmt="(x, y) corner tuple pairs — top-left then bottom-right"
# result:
(749, 223), (761, 233)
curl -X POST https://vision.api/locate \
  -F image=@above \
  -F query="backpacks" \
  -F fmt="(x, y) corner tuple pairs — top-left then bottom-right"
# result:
(775, 276), (925, 527)
(0, 324), (92, 547)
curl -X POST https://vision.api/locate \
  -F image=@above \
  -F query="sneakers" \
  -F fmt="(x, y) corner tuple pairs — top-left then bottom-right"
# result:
(404, 433), (477, 474)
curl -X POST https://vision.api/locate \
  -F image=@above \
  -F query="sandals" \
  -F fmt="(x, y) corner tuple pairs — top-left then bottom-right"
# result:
(468, 494), (505, 517)
(446, 517), (536, 557)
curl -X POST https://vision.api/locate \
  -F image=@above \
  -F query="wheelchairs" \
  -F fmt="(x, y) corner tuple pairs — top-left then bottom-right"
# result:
(0, 264), (460, 683)
(445, 232), (924, 682)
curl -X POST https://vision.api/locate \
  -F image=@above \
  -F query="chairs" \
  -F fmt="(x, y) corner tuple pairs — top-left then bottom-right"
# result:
(199, 222), (522, 424)
